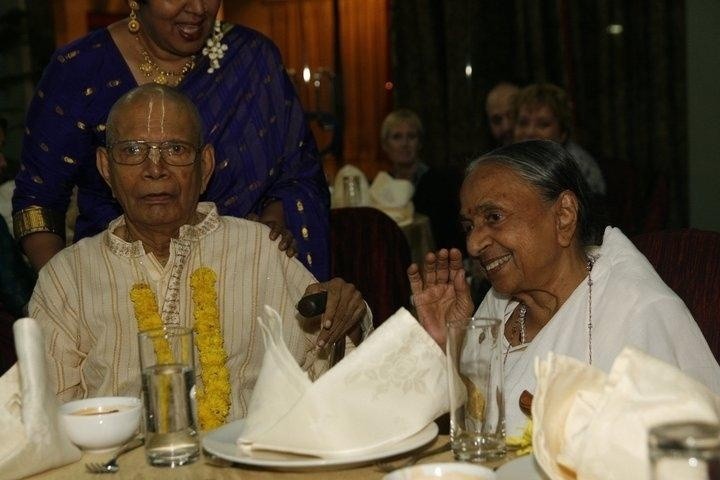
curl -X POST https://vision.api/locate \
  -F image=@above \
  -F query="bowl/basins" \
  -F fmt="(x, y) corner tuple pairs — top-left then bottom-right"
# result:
(57, 396), (141, 453)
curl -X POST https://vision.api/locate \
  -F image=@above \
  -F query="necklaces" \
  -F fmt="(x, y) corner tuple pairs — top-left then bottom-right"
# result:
(131, 33), (195, 86)
(512, 306), (533, 348)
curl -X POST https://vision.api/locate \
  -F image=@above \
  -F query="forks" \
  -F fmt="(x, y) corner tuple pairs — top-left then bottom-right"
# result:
(374, 432), (471, 471)
(85, 438), (145, 472)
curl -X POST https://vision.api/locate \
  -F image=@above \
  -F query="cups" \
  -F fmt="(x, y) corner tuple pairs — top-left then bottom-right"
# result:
(342, 175), (363, 208)
(138, 327), (200, 468)
(447, 318), (507, 462)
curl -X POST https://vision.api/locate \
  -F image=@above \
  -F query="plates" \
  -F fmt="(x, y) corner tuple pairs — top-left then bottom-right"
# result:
(202, 418), (439, 467)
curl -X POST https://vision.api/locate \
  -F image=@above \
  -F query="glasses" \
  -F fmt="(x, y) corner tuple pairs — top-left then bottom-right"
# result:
(106, 139), (202, 166)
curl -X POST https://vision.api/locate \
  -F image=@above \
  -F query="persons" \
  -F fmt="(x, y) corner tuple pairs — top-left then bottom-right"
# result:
(378, 107), (438, 215)
(407, 143), (720, 437)
(485, 80), (521, 145)
(25, 82), (374, 440)
(8, 2), (333, 285)
(514, 83), (607, 196)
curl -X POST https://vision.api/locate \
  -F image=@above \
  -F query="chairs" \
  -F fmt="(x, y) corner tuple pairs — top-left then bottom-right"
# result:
(318, 208), (411, 331)
(628, 229), (720, 363)
